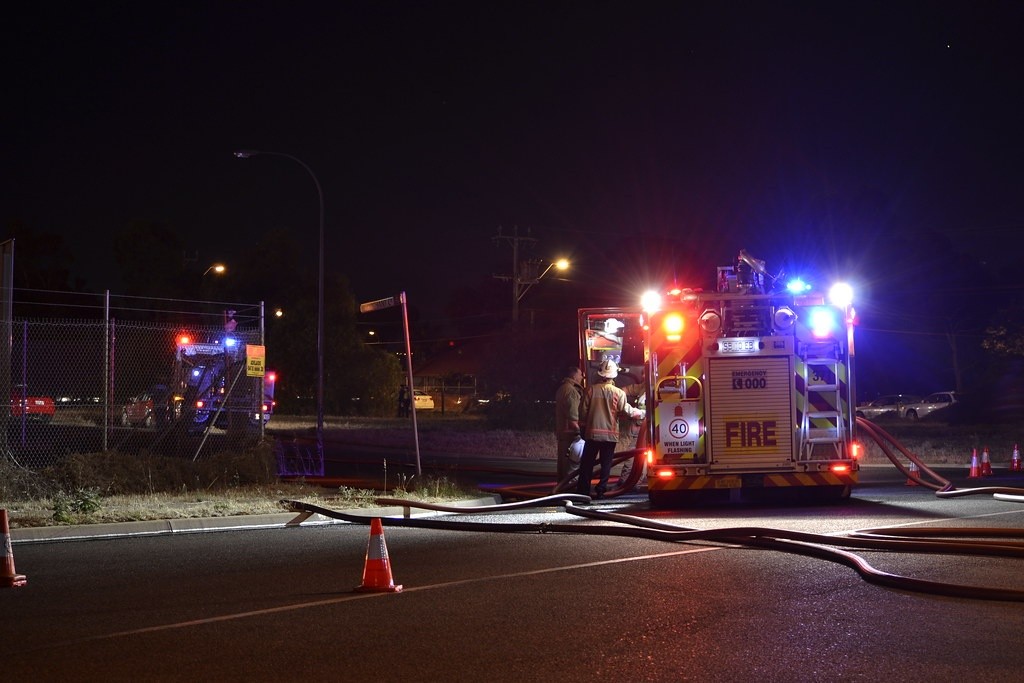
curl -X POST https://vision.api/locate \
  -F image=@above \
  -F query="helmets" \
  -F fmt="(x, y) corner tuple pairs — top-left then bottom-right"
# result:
(568, 439), (585, 463)
(597, 360), (617, 378)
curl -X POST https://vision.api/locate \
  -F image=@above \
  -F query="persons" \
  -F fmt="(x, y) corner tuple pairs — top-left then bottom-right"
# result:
(610, 367), (649, 494)
(554, 367), (585, 491)
(397, 384), (411, 419)
(578, 360), (647, 499)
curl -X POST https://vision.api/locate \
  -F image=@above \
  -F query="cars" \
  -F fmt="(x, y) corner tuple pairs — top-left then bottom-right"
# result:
(408, 389), (435, 411)
(9, 383), (55, 424)
(120, 385), (182, 428)
(900, 390), (960, 421)
(854, 394), (919, 418)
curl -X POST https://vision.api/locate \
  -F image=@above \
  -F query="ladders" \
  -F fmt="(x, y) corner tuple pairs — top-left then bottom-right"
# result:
(799, 341), (849, 459)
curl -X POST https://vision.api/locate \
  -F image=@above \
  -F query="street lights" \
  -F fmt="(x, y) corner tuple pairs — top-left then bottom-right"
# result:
(233, 148), (325, 475)
(513, 260), (568, 322)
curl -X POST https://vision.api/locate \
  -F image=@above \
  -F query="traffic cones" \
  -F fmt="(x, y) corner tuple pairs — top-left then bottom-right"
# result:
(0, 509), (27, 586)
(1009, 443), (1024, 472)
(904, 454), (921, 486)
(980, 447), (993, 475)
(968, 448), (983, 479)
(353, 518), (404, 593)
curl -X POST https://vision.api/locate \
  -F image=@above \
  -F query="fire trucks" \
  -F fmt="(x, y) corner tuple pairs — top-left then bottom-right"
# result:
(576, 250), (863, 511)
(169, 337), (277, 437)
